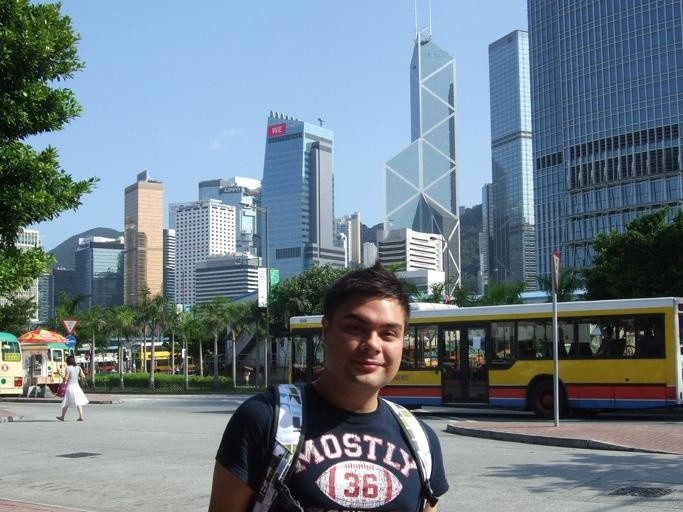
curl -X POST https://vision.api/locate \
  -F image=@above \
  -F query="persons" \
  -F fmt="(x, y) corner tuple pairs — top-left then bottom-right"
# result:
(80, 362), (84, 372)
(595, 326), (614, 356)
(208, 259), (449, 512)
(56, 356), (90, 422)
(244, 369), (251, 386)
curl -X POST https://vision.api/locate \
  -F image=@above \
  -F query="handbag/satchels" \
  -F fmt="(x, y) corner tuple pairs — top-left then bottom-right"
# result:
(57, 383), (66, 397)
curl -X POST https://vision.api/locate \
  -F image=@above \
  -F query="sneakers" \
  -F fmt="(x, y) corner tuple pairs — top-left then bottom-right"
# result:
(77, 418), (84, 421)
(56, 416), (64, 421)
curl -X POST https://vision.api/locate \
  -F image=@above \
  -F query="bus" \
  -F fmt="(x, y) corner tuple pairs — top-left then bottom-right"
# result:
(130, 341), (195, 374)
(66, 344), (130, 374)
(286, 295), (683, 420)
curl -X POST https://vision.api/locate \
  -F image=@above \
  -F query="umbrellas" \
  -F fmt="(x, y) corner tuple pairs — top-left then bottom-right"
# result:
(244, 366), (254, 370)
(20, 327), (66, 397)
